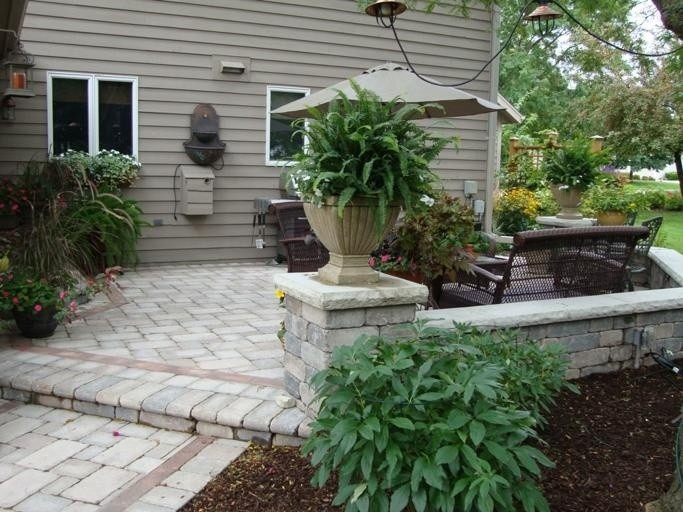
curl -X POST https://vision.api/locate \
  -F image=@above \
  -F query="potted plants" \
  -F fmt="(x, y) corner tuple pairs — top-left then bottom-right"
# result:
(283, 76), (459, 288)
(540, 134), (616, 219)
(590, 189), (630, 226)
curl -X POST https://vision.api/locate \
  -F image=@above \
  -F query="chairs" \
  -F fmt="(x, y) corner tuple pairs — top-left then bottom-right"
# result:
(266, 200), (328, 273)
(431, 216), (664, 304)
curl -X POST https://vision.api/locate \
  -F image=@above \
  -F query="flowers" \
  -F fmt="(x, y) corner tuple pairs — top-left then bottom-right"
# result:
(0, 272), (73, 317)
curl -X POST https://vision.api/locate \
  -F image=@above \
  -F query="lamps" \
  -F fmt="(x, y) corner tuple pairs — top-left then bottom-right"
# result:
(365, 0), (410, 30)
(519, 0), (563, 37)
(0, 28), (38, 121)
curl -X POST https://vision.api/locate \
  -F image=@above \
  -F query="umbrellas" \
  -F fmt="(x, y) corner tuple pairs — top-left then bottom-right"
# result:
(270, 60), (506, 127)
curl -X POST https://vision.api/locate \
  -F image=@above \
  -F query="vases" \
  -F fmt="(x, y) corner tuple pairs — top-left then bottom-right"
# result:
(11, 307), (61, 339)
